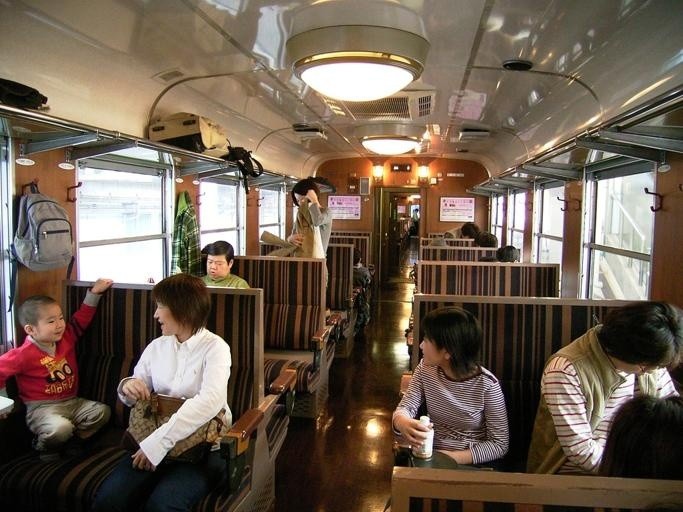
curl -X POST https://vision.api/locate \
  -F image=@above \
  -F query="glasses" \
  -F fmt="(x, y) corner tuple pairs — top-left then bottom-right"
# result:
(639, 366), (655, 376)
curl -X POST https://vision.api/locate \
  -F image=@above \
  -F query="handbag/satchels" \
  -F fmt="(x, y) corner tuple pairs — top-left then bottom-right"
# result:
(121, 392), (227, 467)
(0, 79), (48, 110)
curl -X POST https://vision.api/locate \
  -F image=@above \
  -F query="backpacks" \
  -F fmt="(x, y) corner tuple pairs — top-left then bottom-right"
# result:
(219, 146), (263, 195)
(11, 184), (74, 273)
(164, 112), (232, 161)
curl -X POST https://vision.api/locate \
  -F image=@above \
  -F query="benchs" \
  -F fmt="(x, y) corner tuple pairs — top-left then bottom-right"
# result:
(0, 229), (375, 512)
(390, 232), (683, 511)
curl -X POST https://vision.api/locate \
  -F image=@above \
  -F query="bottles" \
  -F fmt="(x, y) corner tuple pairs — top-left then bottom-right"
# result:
(411, 415), (434, 459)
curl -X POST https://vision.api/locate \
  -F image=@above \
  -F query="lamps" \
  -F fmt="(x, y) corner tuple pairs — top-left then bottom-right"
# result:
(175, 167), (184, 184)
(15, 143), (36, 166)
(658, 160), (671, 172)
(286, 0), (431, 104)
(417, 164), (429, 183)
(353, 121), (427, 156)
(373, 161), (383, 183)
(57, 147), (74, 170)
(192, 174), (200, 185)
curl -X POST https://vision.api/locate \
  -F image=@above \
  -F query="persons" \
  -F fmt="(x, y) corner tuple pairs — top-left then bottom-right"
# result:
(391, 307), (509, 467)
(199, 240), (250, 288)
(352, 247), (370, 287)
(286, 178), (331, 292)
(599, 395), (682, 481)
(0, 278), (112, 451)
(412, 209), (418, 234)
(525, 300), (683, 511)
(93, 273), (233, 511)
(444, 222), (519, 262)
